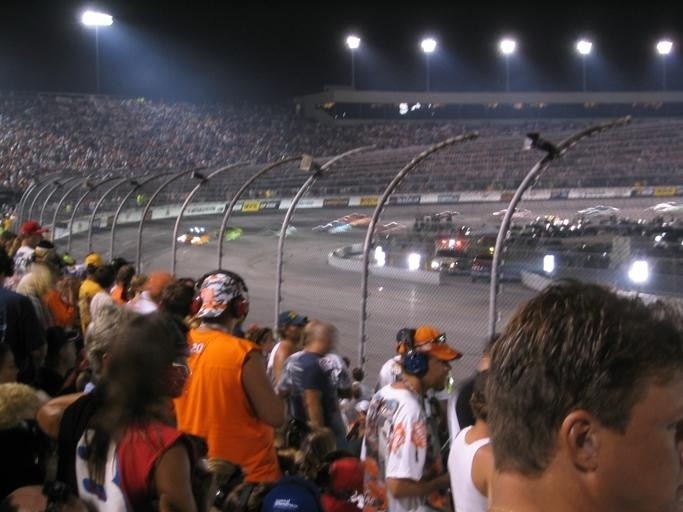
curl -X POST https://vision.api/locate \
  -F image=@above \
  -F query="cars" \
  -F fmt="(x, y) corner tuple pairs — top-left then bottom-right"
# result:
(106, 242), (152, 275)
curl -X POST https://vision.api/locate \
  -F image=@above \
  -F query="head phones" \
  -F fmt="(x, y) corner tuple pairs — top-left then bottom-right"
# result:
(404, 329), (428, 377)
(214, 465), (240, 508)
(240, 484), (257, 512)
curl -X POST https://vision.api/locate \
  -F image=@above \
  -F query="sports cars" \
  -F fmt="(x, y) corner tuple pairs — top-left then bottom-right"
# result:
(311, 220), (351, 235)
(214, 224), (243, 242)
(178, 226), (209, 246)
(262, 214), (295, 241)
(337, 211), (370, 227)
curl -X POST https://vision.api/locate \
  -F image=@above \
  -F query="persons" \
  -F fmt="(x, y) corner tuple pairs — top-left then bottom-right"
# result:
(0, 96), (683, 511)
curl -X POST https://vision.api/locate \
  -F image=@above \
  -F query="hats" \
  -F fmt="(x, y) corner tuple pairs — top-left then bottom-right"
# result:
(46, 324), (80, 352)
(277, 311), (307, 326)
(20, 220), (48, 237)
(400, 326), (463, 362)
(111, 256), (135, 265)
(85, 254), (103, 268)
(263, 473), (322, 511)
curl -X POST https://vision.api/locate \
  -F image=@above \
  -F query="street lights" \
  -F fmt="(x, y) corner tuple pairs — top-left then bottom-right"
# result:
(575, 39), (592, 91)
(420, 37), (437, 92)
(499, 37), (516, 92)
(81, 11), (114, 93)
(345, 34), (361, 90)
(656, 38), (673, 91)
